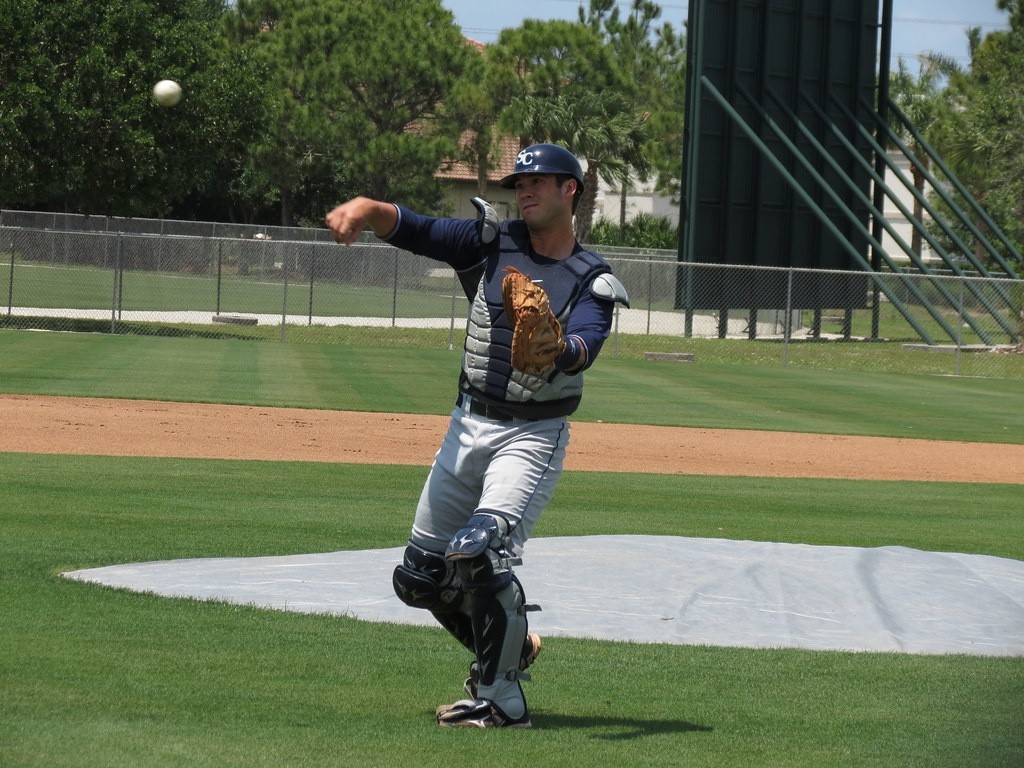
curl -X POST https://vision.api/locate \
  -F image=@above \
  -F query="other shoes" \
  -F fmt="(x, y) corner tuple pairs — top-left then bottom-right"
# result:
(519, 633), (541, 672)
(438, 698), (532, 728)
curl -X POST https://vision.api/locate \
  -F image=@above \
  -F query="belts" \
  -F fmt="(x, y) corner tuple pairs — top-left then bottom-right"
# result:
(456, 391), (538, 421)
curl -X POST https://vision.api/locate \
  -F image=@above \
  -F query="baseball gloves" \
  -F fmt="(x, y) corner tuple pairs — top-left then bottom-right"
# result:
(499, 271), (566, 376)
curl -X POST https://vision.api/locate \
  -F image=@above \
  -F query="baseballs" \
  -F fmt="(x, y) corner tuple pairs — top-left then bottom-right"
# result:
(152, 79), (184, 107)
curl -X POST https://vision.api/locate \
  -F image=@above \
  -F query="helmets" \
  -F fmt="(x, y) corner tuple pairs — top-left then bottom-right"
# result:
(500, 144), (585, 191)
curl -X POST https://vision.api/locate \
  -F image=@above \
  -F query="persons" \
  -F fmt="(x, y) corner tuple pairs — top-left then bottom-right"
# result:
(324, 141), (633, 732)
